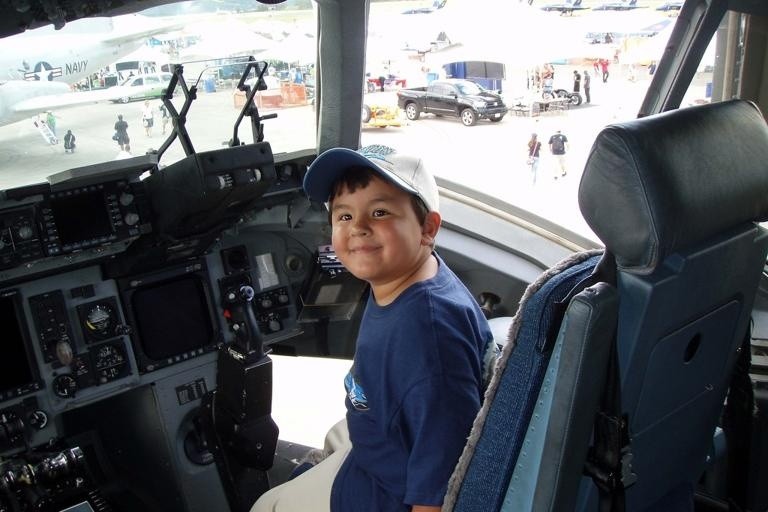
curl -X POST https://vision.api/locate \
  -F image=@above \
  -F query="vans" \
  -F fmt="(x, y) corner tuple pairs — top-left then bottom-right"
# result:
(108, 72), (173, 104)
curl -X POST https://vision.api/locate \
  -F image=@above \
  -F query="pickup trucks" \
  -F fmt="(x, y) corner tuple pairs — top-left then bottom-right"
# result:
(396, 79), (507, 127)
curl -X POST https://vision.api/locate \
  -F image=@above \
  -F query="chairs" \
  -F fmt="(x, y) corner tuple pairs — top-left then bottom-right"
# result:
(442, 98), (768, 512)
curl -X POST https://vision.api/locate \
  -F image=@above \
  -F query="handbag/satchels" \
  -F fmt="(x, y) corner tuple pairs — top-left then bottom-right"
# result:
(112, 132), (121, 140)
(526, 156), (535, 165)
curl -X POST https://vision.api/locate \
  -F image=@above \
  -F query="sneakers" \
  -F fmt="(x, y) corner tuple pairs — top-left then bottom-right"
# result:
(554, 171), (567, 180)
(65, 150), (74, 153)
(147, 131), (167, 138)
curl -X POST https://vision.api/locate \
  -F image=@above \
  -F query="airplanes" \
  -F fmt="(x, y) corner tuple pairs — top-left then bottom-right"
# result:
(0, 25), (183, 144)
(399, 0), (447, 15)
(539, 0), (685, 17)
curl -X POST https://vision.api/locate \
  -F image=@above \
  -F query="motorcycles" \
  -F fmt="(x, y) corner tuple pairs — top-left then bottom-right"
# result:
(542, 85), (582, 106)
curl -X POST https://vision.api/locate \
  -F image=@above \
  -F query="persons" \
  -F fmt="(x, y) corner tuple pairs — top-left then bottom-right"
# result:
(203, 63), (216, 93)
(248, 138), (502, 512)
(605, 32), (614, 43)
(266, 62), (277, 77)
(376, 64), (389, 94)
(646, 59), (656, 80)
(113, 114), (131, 153)
(309, 64), (315, 76)
(527, 131), (542, 181)
(70, 62), (156, 91)
(158, 101), (172, 136)
(47, 110), (56, 135)
(62, 129), (76, 153)
(524, 56), (612, 113)
(546, 129), (568, 180)
(141, 99), (154, 138)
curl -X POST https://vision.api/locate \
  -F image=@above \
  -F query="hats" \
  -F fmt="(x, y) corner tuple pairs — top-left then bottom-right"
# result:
(302, 144), (440, 217)
